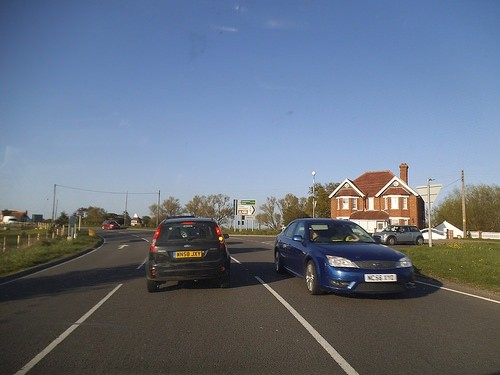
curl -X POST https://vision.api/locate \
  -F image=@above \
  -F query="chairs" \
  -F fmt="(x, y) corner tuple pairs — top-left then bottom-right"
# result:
(298, 230), (320, 241)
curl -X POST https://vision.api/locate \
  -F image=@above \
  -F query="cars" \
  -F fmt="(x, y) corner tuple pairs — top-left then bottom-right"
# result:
(273, 218), (416, 296)
(374, 225), (425, 246)
(101, 220), (120, 230)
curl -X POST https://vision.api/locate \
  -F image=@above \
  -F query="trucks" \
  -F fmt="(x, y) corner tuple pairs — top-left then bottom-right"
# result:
(3, 215), (19, 224)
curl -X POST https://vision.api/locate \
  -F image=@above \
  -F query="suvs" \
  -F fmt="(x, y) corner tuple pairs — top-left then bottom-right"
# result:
(145, 216), (232, 292)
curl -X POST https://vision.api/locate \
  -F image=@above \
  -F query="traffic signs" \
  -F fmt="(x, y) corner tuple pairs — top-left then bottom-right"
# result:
(236, 198), (257, 216)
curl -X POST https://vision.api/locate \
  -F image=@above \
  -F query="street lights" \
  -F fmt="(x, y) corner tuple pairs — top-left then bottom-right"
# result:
(425, 177), (436, 247)
(311, 170), (317, 218)
